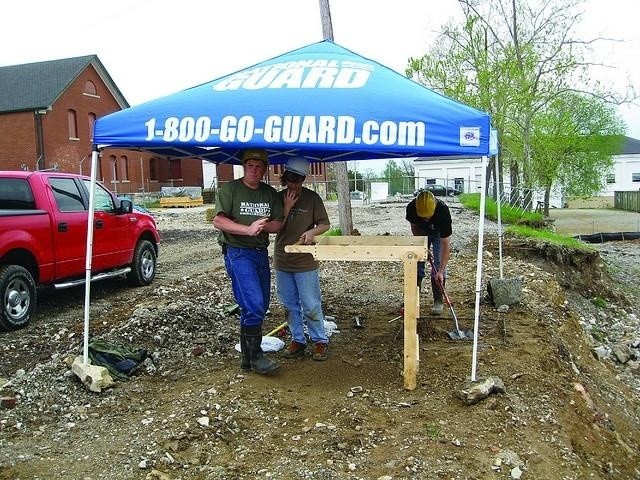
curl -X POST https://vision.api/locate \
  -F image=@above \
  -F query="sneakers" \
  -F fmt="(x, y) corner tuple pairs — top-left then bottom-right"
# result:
(312, 342), (328, 360)
(283, 341), (306, 357)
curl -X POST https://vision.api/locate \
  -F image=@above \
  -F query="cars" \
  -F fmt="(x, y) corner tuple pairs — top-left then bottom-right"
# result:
(414, 184), (462, 197)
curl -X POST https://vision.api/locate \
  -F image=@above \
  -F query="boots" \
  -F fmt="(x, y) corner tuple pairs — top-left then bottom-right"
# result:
(240, 324), (279, 374)
(417, 275), (445, 315)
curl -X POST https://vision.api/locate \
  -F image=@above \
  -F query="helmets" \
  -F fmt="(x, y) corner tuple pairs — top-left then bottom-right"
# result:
(286, 156), (309, 176)
(415, 191), (436, 218)
(241, 149), (268, 164)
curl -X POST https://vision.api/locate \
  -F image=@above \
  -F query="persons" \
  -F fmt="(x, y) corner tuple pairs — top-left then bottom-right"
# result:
(212, 149), (284, 374)
(401, 189), (452, 315)
(274, 156), (329, 361)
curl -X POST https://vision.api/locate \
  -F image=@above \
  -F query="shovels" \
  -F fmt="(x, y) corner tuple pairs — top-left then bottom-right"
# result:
(427, 253), (474, 340)
(352, 316), (362, 328)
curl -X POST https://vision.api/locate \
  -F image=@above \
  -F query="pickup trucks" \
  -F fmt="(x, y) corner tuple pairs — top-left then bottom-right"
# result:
(0, 166), (160, 331)
